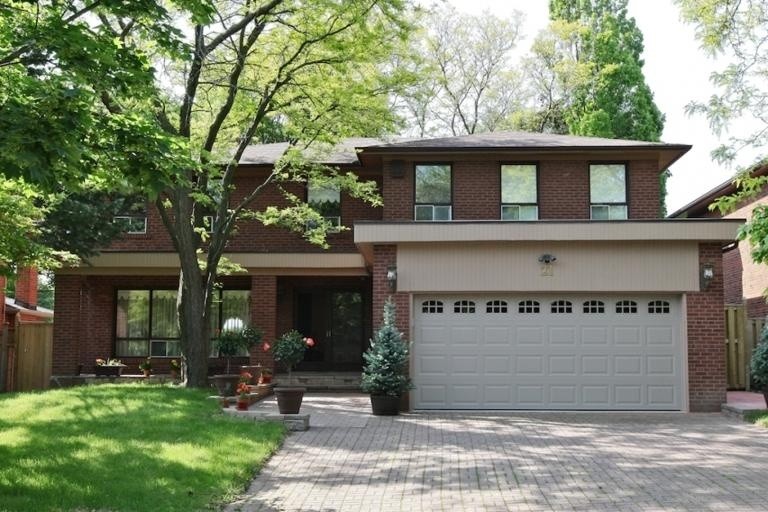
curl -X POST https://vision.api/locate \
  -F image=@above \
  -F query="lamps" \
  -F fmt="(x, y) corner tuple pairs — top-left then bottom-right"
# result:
(537, 254), (556, 263)
(385, 265), (396, 290)
(698, 263), (713, 292)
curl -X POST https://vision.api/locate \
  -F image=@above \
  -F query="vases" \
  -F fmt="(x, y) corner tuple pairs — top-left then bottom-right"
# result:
(270, 380), (309, 416)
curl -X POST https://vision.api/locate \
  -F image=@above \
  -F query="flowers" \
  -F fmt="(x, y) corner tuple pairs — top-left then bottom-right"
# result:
(263, 328), (314, 387)
(94, 355), (181, 379)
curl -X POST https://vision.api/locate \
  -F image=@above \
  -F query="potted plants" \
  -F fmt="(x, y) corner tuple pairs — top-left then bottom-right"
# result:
(206, 322), (262, 412)
(358, 293), (418, 417)
